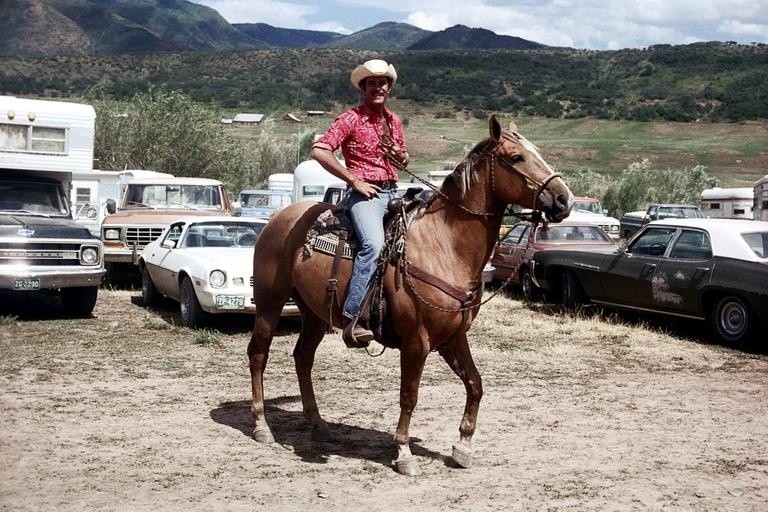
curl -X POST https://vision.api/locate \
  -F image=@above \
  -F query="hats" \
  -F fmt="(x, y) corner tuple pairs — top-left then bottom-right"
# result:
(351, 60), (397, 90)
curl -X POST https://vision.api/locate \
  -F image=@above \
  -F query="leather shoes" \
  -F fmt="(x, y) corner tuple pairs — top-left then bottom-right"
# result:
(340, 318), (374, 348)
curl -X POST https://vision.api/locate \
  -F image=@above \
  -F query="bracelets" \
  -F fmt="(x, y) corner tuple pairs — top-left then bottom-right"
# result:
(401, 153), (407, 165)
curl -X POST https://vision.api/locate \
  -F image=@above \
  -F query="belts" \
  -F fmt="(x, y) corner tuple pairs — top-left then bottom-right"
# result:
(370, 180), (397, 189)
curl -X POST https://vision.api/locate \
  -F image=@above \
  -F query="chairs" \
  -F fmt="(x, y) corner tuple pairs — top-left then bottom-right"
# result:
(186, 234), (207, 247)
(697, 246), (711, 259)
(676, 243), (696, 260)
(234, 232), (257, 246)
(19, 191), (52, 208)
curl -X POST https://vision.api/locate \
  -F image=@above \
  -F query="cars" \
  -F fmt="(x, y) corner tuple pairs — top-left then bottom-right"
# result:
(574, 196), (609, 217)
(136, 217), (301, 328)
(518, 204), (622, 241)
(490, 220), (621, 302)
(531, 217), (768, 346)
(232, 160), (352, 216)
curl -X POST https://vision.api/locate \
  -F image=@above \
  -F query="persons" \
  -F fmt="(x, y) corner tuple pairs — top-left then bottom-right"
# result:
(314, 60), (409, 343)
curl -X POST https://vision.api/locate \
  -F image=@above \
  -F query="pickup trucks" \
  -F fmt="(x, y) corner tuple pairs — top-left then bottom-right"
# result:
(618, 203), (706, 244)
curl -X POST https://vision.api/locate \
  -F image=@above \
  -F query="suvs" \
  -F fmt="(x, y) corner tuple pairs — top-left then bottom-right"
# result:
(100, 177), (233, 288)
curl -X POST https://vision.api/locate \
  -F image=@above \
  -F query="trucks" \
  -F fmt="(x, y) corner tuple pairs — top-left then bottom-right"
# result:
(0, 96), (108, 315)
(70, 169), (184, 236)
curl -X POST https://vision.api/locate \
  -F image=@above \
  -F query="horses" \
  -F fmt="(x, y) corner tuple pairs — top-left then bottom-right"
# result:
(246, 112), (577, 478)
